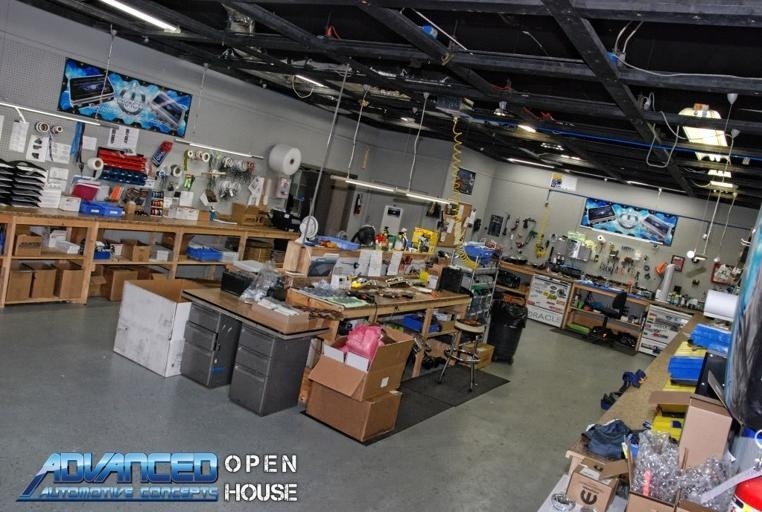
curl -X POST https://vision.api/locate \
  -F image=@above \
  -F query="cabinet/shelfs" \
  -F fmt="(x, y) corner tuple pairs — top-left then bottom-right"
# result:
(215, 257), (472, 409)
(489, 254), (702, 357)
(0, 201), (305, 306)
(444, 259), (499, 364)
(564, 303), (735, 485)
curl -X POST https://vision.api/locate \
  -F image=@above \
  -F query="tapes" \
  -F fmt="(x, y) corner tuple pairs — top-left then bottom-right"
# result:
(222, 180), (241, 195)
(183, 149), (247, 173)
(51, 126), (63, 134)
(171, 165), (183, 177)
(34, 122), (49, 133)
(597, 235), (606, 244)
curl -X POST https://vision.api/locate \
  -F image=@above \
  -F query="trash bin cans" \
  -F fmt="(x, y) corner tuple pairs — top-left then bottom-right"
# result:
(487, 300), (528, 366)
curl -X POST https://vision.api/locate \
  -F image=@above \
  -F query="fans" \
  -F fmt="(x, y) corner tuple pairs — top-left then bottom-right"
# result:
(292, 213), (321, 246)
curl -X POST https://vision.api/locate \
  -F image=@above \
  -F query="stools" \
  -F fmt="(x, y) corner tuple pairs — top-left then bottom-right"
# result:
(436, 318), (486, 395)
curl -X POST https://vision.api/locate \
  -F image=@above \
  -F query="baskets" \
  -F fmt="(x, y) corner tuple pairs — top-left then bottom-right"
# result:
(229, 236), (273, 265)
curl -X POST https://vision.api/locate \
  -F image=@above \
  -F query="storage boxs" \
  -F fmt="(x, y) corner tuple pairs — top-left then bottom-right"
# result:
(35, 184), (107, 217)
(458, 340), (496, 371)
(559, 353), (734, 511)
(0, 204), (280, 303)
(108, 272), (418, 445)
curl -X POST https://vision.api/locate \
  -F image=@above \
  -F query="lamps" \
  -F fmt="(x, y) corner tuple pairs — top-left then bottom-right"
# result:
(327, 84), (457, 212)
(660, 88), (742, 203)
(94, 0), (184, 36)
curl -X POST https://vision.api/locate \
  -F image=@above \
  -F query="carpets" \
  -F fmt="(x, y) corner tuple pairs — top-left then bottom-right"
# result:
(399, 359), (510, 409)
(297, 365), (454, 447)
